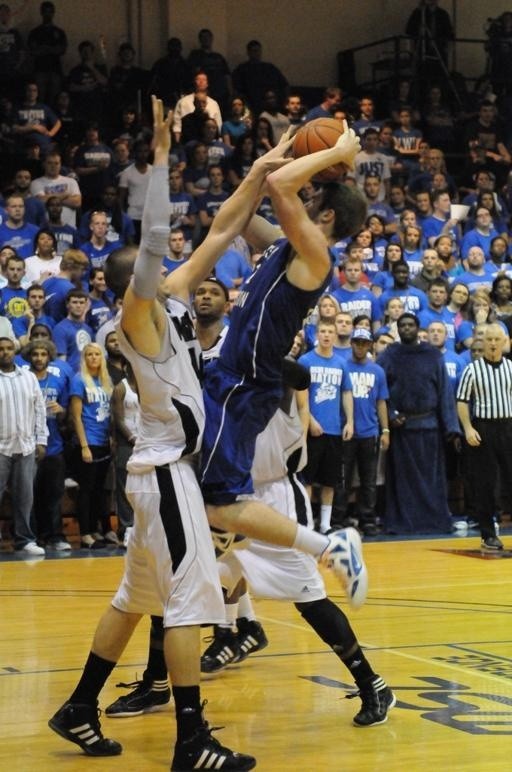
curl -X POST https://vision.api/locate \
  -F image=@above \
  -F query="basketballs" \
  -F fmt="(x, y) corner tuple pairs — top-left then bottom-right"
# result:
(292, 116), (346, 185)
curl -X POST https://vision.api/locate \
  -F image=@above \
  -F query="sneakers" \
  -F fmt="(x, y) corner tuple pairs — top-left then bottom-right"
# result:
(47, 699), (122, 755)
(13, 542), (45, 555)
(357, 515), (377, 536)
(452, 514), (479, 530)
(201, 624), (240, 672)
(321, 527), (368, 607)
(170, 727), (256, 772)
(353, 674), (397, 728)
(481, 537), (503, 550)
(236, 617), (267, 662)
(105, 671), (170, 717)
(47, 540), (71, 551)
(81, 531), (119, 549)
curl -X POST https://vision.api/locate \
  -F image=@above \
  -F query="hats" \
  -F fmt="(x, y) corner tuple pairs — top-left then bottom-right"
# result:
(20, 339), (57, 362)
(351, 327), (373, 342)
(374, 327), (396, 343)
